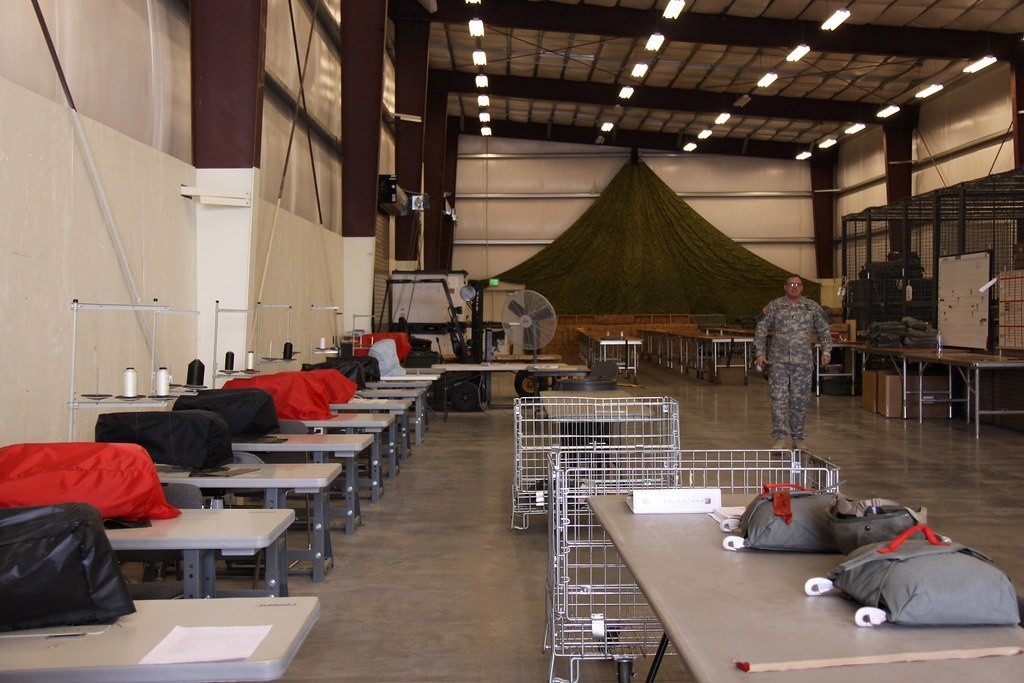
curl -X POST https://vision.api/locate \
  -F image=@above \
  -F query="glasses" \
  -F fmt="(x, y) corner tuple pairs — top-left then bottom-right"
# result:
(785, 282), (802, 288)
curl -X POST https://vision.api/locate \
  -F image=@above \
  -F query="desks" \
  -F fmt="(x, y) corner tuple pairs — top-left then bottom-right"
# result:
(0, 324), (1023, 683)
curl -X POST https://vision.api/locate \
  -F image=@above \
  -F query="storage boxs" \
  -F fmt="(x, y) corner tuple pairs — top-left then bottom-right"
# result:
(862, 369), (916, 413)
(877, 368), (956, 417)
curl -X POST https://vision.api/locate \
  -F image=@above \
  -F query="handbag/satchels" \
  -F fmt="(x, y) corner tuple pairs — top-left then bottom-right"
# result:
(826, 522), (1021, 626)
(823, 490), (930, 555)
(738, 479), (846, 553)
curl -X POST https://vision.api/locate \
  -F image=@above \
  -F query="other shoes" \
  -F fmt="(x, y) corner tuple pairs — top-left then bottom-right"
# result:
(773, 438), (789, 456)
(793, 438), (806, 451)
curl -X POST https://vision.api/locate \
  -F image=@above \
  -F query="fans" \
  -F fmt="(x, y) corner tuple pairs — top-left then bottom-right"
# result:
(499, 290), (558, 359)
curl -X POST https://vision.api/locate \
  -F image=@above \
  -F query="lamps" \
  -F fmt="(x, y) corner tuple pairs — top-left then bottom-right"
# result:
(846, 123), (868, 136)
(714, 112), (730, 125)
(465, 0), (492, 137)
(646, 33), (665, 53)
(735, 94), (751, 108)
(915, 81), (944, 100)
(822, 8), (850, 33)
(757, 72), (779, 89)
(962, 55), (997, 75)
(602, 84), (635, 132)
(697, 129), (712, 139)
(631, 62), (650, 79)
(877, 105), (903, 118)
(797, 150), (811, 162)
(819, 135), (838, 149)
(785, 42), (812, 62)
(683, 143), (697, 153)
(661, 0), (686, 20)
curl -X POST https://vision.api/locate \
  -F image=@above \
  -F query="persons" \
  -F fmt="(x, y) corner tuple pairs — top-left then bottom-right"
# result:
(754, 275), (833, 455)
